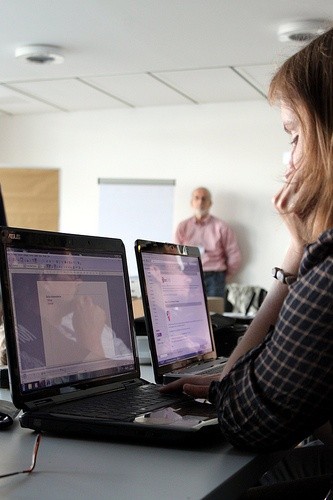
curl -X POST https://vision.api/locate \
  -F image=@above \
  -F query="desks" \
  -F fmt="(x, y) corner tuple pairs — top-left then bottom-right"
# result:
(0, 365), (260, 500)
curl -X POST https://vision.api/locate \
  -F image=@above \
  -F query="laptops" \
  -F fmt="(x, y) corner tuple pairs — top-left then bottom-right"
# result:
(135, 239), (229, 386)
(0, 226), (223, 445)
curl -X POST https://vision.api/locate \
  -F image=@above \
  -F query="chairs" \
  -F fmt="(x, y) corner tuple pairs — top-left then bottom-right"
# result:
(221, 283), (267, 328)
